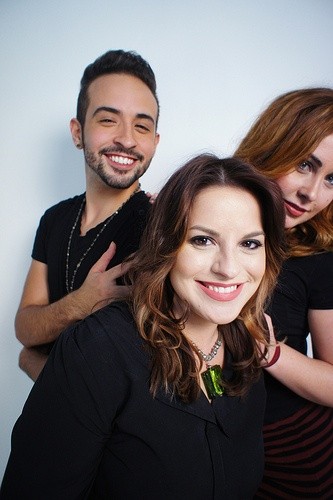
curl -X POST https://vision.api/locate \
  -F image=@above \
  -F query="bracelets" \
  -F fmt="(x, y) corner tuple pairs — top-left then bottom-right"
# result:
(263, 341), (280, 369)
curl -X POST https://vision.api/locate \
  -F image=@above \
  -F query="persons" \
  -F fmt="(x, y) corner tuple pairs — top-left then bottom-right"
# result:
(0, 153), (289, 500)
(145, 87), (333, 500)
(14, 50), (160, 384)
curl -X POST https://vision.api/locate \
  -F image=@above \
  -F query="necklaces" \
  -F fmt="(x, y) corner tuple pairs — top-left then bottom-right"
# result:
(65, 183), (141, 293)
(188, 336), (225, 399)
